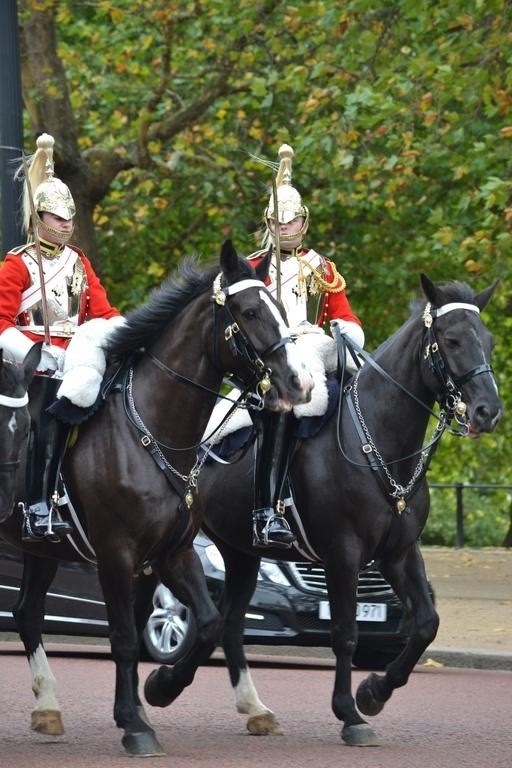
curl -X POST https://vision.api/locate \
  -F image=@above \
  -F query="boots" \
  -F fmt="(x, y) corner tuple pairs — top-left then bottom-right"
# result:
(20, 375), (73, 542)
(252, 408), (298, 547)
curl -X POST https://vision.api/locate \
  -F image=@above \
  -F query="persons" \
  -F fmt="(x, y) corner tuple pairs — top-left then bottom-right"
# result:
(1, 209), (131, 542)
(241, 213), (365, 548)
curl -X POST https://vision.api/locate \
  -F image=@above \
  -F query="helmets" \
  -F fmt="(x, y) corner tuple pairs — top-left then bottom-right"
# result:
(33, 175), (77, 244)
(264, 186), (310, 250)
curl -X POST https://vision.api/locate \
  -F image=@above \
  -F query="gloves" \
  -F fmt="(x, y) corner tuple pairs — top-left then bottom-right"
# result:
(41, 342), (64, 368)
(330, 318), (351, 342)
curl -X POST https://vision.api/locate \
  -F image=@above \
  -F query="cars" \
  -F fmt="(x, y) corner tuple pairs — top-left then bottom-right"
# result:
(0, 517), (440, 674)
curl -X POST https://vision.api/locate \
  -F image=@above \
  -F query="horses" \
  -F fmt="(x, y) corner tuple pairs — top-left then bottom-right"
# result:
(0, 233), (305, 760)
(135, 269), (504, 751)
